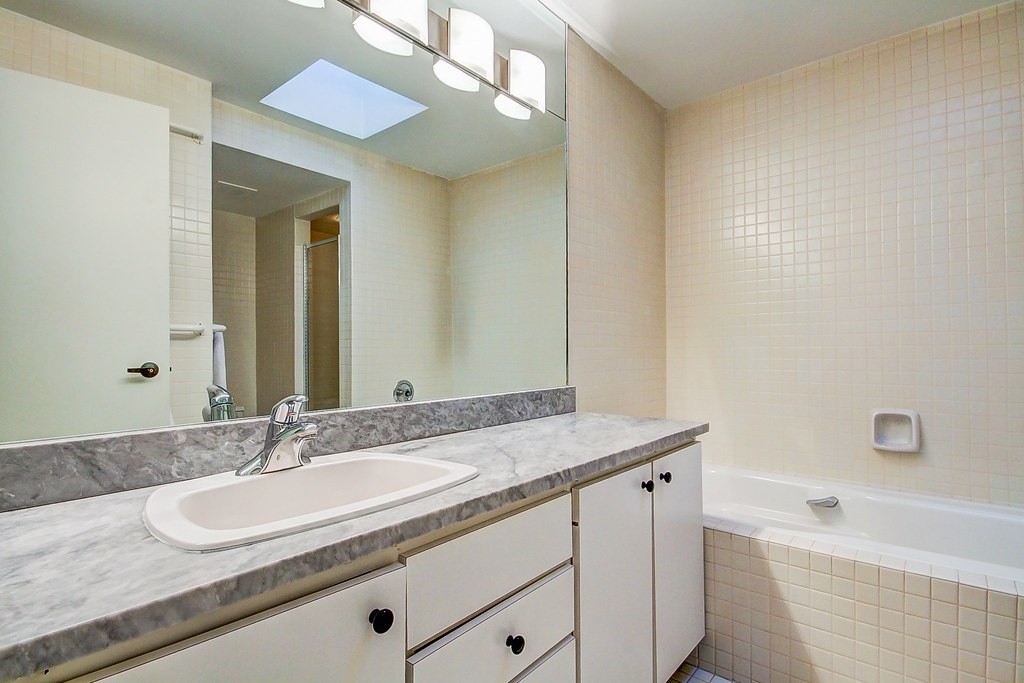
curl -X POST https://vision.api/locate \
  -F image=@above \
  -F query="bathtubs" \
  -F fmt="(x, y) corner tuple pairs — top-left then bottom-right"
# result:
(698, 465), (1023, 683)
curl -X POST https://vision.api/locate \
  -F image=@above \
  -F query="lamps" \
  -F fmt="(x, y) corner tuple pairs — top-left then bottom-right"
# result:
(506, 49), (547, 114)
(494, 90), (532, 121)
(432, 55), (480, 93)
(351, 10), (413, 57)
(447, 7), (495, 86)
(359, 0), (429, 47)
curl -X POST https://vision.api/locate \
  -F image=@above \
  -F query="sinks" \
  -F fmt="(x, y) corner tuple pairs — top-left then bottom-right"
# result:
(143, 450), (478, 553)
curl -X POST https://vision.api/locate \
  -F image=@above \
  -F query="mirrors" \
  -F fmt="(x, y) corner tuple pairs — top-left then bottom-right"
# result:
(0, 0), (570, 447)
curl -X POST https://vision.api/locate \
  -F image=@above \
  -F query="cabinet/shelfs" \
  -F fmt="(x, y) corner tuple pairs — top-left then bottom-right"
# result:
(565, 438), (705, 683)
(12, 483), (577, 683)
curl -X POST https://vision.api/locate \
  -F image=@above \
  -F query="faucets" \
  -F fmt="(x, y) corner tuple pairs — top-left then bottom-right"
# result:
(202, 384), (235, 422)
(237, 396), (319, 475)
